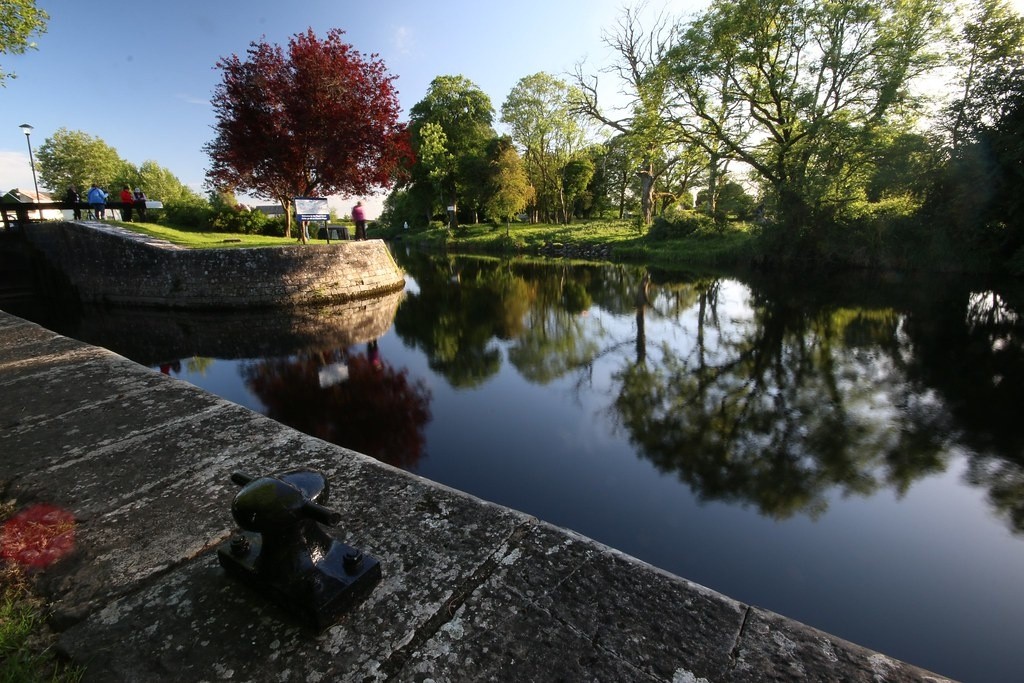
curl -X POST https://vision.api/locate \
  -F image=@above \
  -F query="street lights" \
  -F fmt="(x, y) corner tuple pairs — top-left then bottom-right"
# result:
(19, 124), (44, 220)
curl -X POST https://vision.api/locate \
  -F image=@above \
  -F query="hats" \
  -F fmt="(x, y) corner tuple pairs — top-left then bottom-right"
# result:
(135, 188), (140, 193)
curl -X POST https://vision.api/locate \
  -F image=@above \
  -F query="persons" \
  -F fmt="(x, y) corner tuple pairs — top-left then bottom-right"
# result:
(160, 362), (180, 375)
(369, 340), (382, 372)
(120, 186), (134, 221)
(68, 184), (81, 220)
(88, 184), (108, 220)
(297, 221), (310, 241)
(352, 201), (367, 240)
(133, 188), (146, 222)
